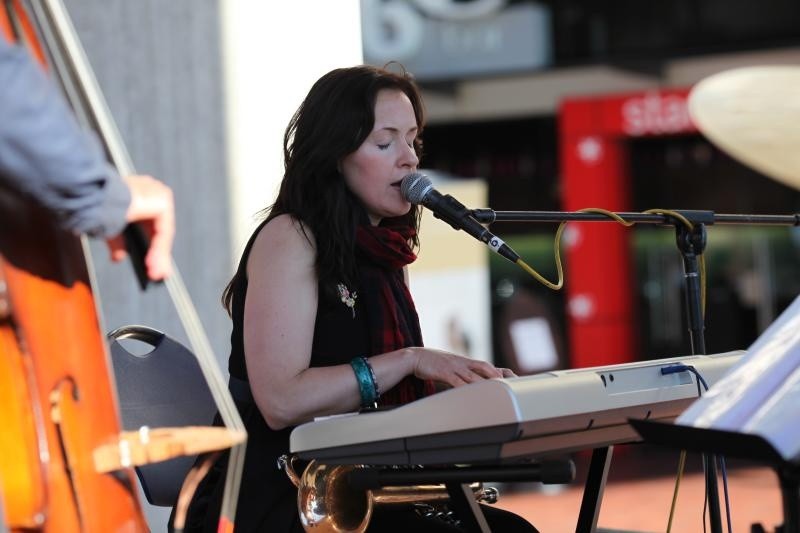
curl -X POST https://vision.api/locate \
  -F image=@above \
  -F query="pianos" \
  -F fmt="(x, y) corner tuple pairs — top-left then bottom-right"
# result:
(288, 347), (753, 533)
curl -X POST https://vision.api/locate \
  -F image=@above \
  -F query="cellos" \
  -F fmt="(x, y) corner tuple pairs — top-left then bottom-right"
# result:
(1, 0), (251, 533)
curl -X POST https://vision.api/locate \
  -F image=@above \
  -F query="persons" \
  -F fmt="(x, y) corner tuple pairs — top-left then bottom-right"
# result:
(1, 34), (175, 284)
(167, 67), (539, 533)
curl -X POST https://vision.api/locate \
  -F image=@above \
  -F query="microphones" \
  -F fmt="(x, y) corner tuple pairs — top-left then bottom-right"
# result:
(401, 173), (519, 262)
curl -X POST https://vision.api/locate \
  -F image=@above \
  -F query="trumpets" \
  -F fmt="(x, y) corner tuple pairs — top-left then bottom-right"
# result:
(283, 455), (501, 533)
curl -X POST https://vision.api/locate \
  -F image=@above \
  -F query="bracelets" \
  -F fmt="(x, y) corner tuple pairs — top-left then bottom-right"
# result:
(349, 357), (380, 401)
